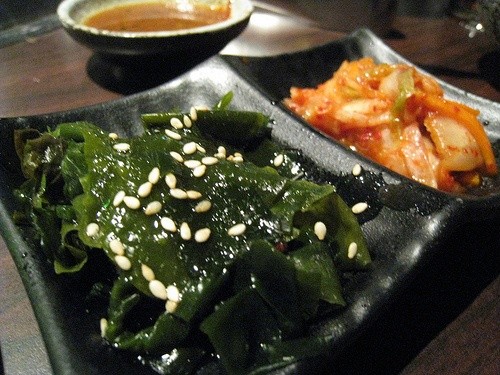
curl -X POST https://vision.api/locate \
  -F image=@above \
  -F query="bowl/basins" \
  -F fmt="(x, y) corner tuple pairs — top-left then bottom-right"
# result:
(56, 0), (253, 60)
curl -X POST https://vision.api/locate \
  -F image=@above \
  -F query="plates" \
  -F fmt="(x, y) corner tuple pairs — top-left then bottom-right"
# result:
(234, 28), (500, 213)
(0, 56), (463, 374)
